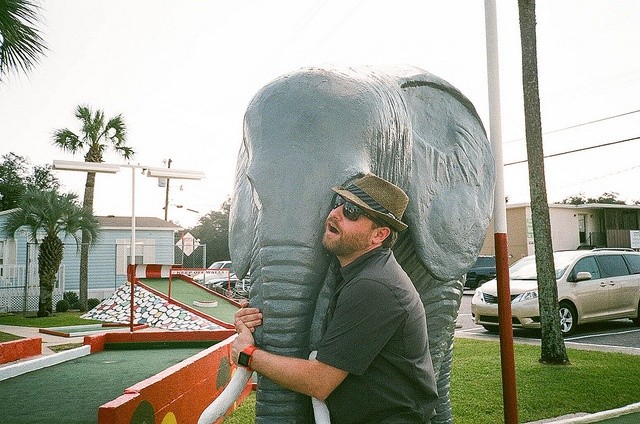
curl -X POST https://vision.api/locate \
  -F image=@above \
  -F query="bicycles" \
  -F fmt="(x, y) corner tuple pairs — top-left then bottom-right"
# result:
(225, 286), (248, 297)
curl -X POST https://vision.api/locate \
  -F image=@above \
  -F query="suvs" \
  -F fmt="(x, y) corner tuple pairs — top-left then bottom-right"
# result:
(193, 260), (250, 283)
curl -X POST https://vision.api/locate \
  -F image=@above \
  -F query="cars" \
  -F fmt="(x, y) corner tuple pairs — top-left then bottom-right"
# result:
(205, 273), (250, 289)
(464, 255), (497, 289)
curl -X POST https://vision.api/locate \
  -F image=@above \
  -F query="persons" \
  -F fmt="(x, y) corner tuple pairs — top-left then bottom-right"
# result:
(232, 172), (440, 424)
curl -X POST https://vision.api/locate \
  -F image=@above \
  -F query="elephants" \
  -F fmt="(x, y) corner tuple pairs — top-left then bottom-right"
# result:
(198, 64), (496, 424)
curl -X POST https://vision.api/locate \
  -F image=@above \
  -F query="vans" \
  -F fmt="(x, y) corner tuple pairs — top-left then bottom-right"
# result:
(471, 246), (640, 336)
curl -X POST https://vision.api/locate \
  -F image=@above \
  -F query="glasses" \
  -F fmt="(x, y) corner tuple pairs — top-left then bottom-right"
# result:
(331, 192), (383, 227)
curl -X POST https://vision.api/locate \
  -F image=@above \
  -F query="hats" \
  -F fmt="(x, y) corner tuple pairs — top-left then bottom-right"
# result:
(331, 171), (410, 232)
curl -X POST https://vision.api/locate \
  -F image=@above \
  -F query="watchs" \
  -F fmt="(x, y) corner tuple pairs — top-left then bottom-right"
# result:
(235, 345), (259, 368)
